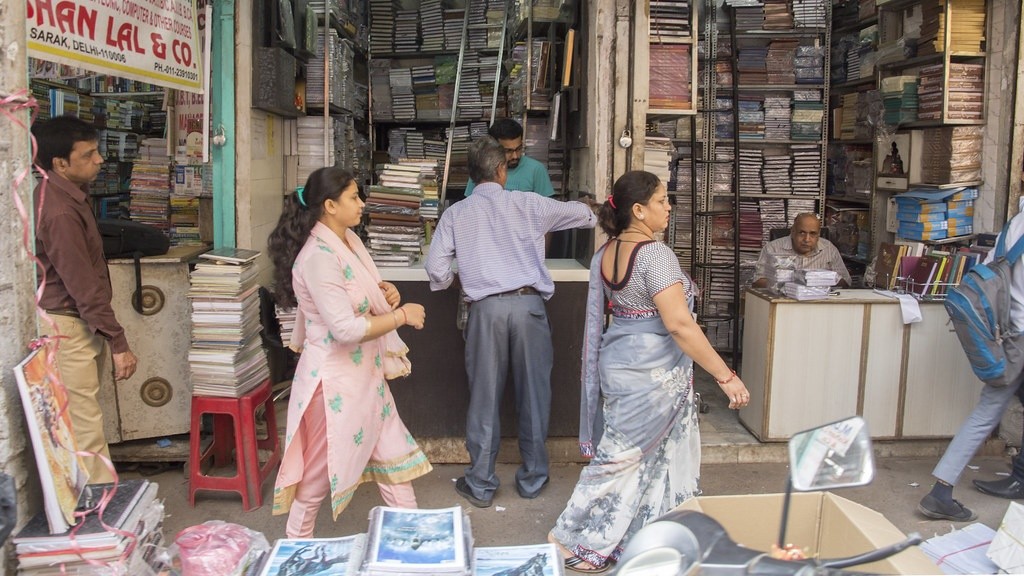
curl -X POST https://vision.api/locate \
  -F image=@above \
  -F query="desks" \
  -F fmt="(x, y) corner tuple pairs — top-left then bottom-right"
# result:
(735, 288), (986, 442)
(103, 242), (213, 448)
(193, 192), (215, 242)
(371, 257), (589, 438)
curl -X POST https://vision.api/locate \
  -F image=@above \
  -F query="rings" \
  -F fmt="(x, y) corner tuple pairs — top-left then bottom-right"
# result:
(730, 398), (736, 404)
(741, 394), (747, 397)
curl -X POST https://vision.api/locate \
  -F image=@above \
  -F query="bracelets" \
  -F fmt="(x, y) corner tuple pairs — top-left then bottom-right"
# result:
(398, 307), (408, 325)
(392, 310), (399, 330)
(714, 368), (737, 384)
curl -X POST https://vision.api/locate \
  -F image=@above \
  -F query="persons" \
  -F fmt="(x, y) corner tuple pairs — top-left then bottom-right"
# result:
(425, 132), (598, 511)
(752, 212), (852, 288)
(547, 172), (750, 573)
(32, 116), (138, 484)
(919, 195), (1024, 522)
(267, 165), (432, 538)
(973, 371), (1024, 498)
(463, 118), (556, 258)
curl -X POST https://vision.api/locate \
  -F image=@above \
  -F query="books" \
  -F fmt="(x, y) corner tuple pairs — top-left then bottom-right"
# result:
(645, 0), (838, 263)
(919, 502), (1024, 575)
(12, 480), (562, 576)
(274, 303), (297, 348)
(679, 263), (758, 350)
(872, 235), (999, 300)
(188, 245), (269, 399)
(31, 73), (200, 245)
(828, 0), (986, 241)
(794, 267), (837, 287)
(284, 0), (579, 268)
(782, 282), (831, 302)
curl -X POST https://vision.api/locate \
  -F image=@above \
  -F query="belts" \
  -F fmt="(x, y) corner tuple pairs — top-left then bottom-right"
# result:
(489, 287), (541, 296)
(46, 307), (81, 319)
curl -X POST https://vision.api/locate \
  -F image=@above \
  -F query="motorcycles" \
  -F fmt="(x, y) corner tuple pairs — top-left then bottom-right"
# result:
(604, 416), (925, 576)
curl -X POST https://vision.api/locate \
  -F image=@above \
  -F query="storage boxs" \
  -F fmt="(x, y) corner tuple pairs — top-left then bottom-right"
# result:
(647, 489), (941, 576)
(896, 189), (978, 242)
(887, 124), (988, 246)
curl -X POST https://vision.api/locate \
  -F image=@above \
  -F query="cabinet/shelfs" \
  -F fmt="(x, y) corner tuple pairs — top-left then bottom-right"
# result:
(826, 0), (893, 265)
(641, 1), (699, 116)
(704, 0), (834, 356)
(643, 1), (711, 320)
(500, 1), (581, 257)
(27, 67), (171, 220)
(282, 1), (368, 196)
(882, 0), (987, 129)
(369, 47), (513, 190)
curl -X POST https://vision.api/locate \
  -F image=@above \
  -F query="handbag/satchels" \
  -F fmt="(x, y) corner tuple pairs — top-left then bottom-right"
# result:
(95, 218), (169, 258)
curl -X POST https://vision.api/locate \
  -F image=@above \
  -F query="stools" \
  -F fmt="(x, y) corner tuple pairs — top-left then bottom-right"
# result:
(187, 381), (280, 512)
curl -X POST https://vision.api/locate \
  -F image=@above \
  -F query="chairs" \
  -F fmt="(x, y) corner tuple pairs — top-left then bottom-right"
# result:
(769, 226), (829, 243)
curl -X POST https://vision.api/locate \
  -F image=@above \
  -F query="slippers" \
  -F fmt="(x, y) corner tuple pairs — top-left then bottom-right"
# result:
(564, 555), (611, 573)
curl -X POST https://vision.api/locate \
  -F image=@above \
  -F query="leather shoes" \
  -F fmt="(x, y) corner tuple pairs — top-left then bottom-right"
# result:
(972, 475), (1024, 500)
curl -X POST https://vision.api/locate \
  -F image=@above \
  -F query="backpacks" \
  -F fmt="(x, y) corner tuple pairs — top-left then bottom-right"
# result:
(943, 217), (1024, 387)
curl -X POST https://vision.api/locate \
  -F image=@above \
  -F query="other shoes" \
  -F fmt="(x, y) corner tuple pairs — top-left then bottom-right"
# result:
(455, 475), (492, 508)
(541, 476), (550, 486)
(918, 494), (978, 521)
(77, 502), (102, 516)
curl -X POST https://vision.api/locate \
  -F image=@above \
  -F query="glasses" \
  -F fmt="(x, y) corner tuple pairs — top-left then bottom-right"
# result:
(503, 142), (527, 154)
